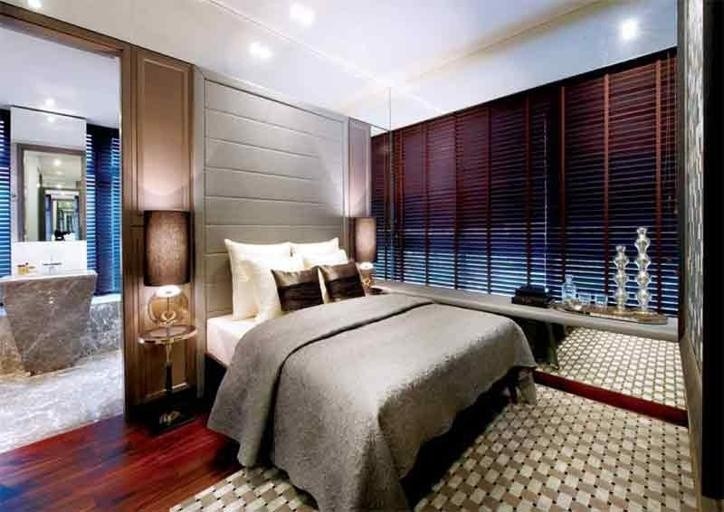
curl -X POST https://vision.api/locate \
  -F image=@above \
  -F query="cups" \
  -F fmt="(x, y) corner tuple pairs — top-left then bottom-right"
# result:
(577, 292), (591, 312)
(595, 294), (608, 314)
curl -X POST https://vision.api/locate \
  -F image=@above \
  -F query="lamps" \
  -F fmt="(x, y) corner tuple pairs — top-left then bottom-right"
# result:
(348, 215), (378, 291)
(140, 208), (193, 329)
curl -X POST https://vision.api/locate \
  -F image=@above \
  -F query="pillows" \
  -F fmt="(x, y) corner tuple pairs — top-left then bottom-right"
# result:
(224, 233), (367, 324)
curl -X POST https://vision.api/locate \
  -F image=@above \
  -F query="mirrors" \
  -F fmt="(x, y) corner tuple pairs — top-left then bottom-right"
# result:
(516, 318), (687, 409)
(14, 142), (86, 243)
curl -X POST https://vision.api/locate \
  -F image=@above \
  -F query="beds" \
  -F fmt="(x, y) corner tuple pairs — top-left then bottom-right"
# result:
(202, 292), (525, 511)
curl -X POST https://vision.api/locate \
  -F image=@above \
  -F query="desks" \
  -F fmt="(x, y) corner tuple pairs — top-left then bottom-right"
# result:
(136, 325), (196, 434)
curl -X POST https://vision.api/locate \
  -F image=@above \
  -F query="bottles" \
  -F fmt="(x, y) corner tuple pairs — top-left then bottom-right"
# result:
(562, 273), (575, 307)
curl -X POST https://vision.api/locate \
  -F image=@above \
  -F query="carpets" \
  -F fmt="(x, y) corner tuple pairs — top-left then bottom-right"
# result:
(535, 328), (686, 409)
(168, 383), (700, 512)
